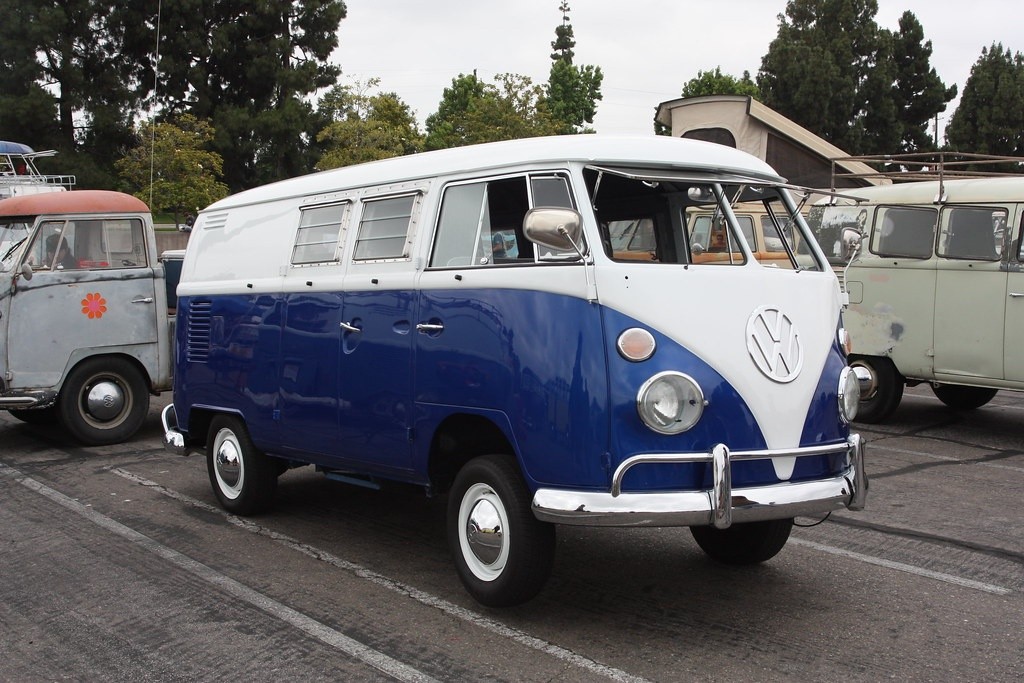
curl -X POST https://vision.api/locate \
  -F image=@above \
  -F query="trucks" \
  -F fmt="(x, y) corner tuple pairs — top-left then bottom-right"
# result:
(0, 187), (188, 446)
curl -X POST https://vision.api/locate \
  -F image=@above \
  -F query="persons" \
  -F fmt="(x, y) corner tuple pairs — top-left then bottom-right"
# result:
(43, 234), (80, 269)
(712, 235), (726, 248)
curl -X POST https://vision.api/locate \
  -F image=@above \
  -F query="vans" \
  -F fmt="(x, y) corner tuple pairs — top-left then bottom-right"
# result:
(158, 132), (872, 611)
(788, 148), (1024, 424)
(1, 137), (79, 272)
(606, 202), (811, 271)
